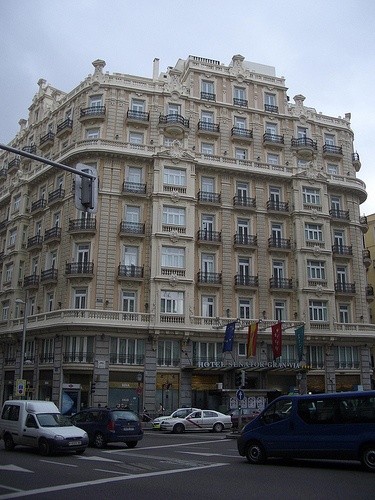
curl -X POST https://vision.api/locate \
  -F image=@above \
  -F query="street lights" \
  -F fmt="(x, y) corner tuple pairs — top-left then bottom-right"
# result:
(14, 289), (29, 379)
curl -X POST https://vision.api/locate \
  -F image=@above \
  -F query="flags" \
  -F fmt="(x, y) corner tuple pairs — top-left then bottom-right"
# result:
(294, 325), (304, 362)
(272, 322), (282, 357)
(223, 322), (235, 353)
(247, 322), (258, 358)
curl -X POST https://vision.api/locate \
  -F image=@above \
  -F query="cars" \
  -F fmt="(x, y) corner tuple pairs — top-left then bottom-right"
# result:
(68, 407), (144, 449)
(151, 408), (233, 434)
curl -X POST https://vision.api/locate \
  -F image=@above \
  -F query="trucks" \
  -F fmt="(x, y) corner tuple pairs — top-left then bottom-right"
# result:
(0, 400), (89, 457)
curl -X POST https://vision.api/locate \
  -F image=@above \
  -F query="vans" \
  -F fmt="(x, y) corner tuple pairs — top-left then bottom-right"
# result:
(237, 390), (375, 473)
